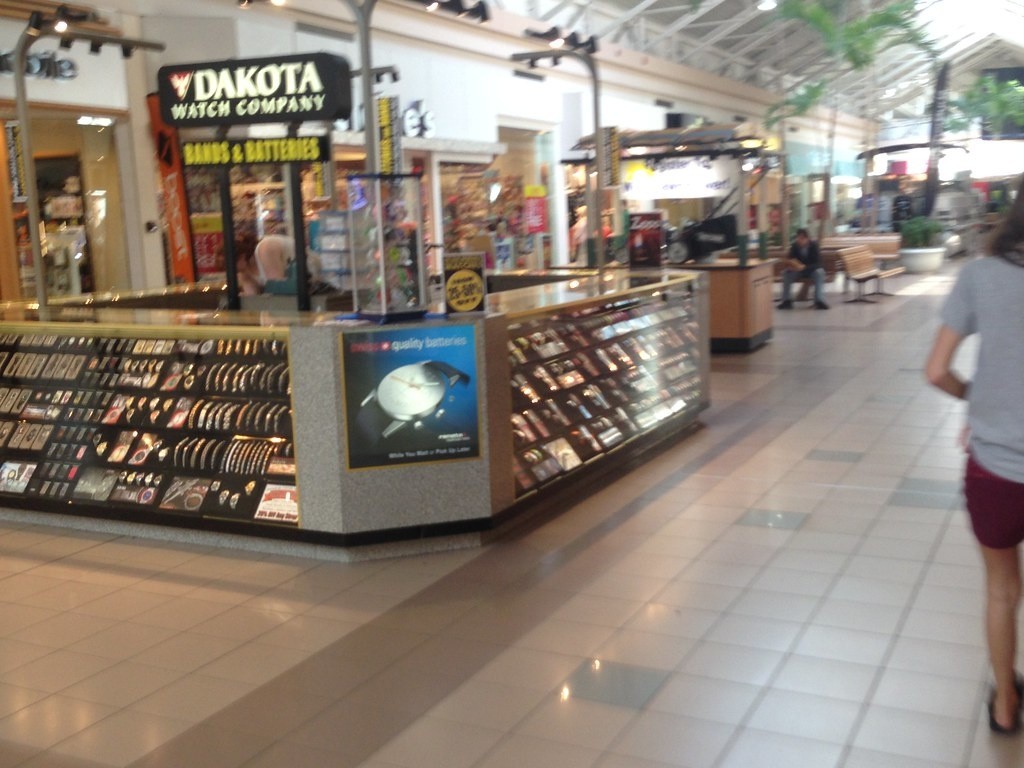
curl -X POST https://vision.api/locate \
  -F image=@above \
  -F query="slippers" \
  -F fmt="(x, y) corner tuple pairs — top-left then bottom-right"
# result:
(988, 688), (1017, 734)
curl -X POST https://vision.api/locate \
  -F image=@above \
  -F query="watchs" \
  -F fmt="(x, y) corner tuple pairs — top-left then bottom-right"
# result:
(356, 358), (470, 448)
(0, 333), (291, 512)
(509, 291), (697, 479)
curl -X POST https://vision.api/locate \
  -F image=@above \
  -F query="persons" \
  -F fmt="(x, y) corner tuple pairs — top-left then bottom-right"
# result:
(258, 217), (320, 297)
(493, 217), (514, 271)
(924, 178), (1024, 734)
(780, 229), (828, 310)
(568, 205), (587, 262)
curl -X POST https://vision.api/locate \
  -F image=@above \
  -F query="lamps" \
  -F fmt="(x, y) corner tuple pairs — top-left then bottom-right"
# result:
(527, 27), (564, 49)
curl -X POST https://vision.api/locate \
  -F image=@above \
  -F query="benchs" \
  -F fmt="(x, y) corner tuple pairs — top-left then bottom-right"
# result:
(721, 252), (835, 301)
(838, 245), (906, 301)
(816, 234), (903, 270)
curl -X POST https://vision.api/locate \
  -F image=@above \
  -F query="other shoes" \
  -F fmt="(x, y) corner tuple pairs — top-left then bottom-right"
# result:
(776, 300), (795, 310)
(814, 300), (828, 308)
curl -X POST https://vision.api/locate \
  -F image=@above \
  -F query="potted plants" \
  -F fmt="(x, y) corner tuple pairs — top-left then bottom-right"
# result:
(898, 216), (945, 273)
